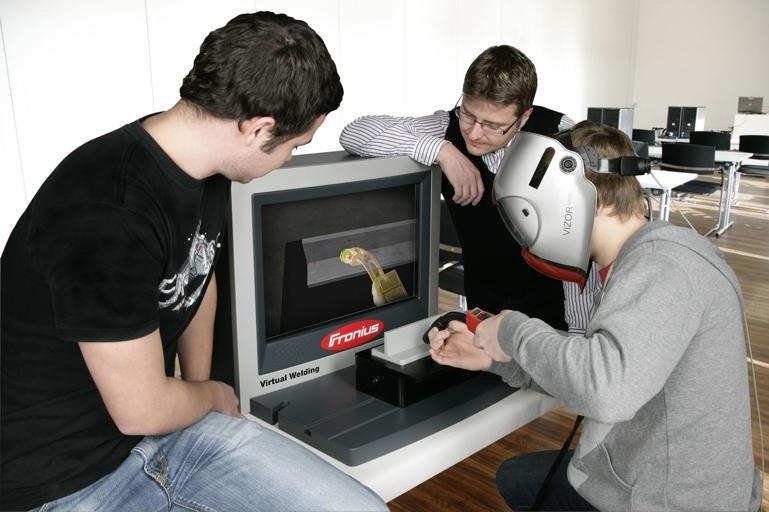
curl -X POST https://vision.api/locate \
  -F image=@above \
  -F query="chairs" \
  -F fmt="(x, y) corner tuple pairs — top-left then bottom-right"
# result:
(629, 128), (769, 239)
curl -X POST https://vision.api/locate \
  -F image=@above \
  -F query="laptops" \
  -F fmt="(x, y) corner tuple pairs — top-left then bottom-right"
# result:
(738, 96), (763, 115)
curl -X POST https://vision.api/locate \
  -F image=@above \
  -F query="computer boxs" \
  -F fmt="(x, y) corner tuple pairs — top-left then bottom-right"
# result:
(586, 106), (634, 143)
(666, 105), (706, 139)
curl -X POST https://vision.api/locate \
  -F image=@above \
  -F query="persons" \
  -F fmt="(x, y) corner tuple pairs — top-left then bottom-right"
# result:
(0, 7), (399, 512)
(338, 44), (604, 339)
(425, 119), (764, 512)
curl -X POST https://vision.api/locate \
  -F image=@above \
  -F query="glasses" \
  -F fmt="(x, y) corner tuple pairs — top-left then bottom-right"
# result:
(455, 93), (521, 137)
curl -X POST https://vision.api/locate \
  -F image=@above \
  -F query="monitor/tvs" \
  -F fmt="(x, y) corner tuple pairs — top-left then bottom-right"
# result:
(198, 151), (442, 399)
